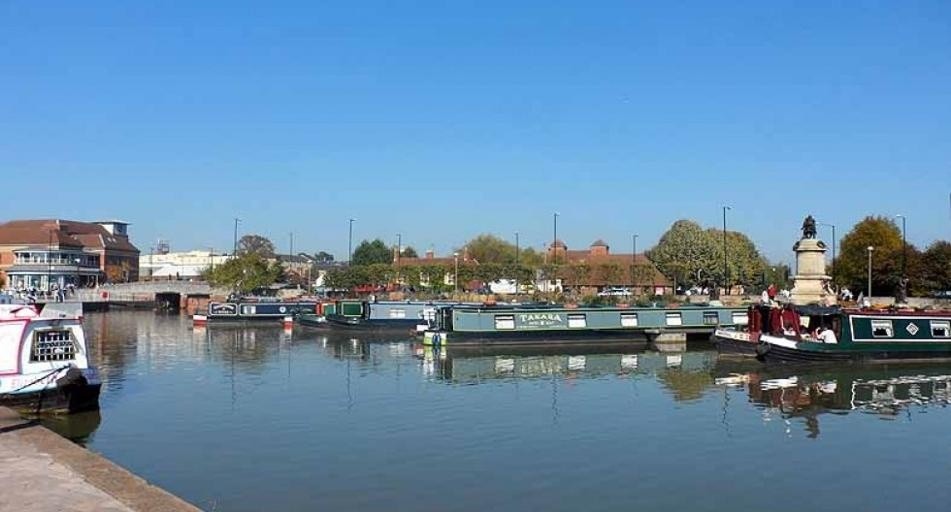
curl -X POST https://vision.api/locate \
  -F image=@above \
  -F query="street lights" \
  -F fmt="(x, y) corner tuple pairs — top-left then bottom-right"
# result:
(346, 218), (356, 267)
(551, 210), (560, 294)
(865, 244), (875, 304)
(287, 230), (297, 262)
(47, 228), (57, 293)
(816, 221), (838, 295)
(393, 233), (402, 268)
(632, 232), (641, 294)
(894, 213), (908, 277)
(234, 215), (243, 260)
(75, 257), (81, 289)
(512, 232), (520, 294)
(721, 202), (735, 297)
(206, 246), (214, 273)
(148, 246), (154, 281)
(451, 253), (460, 295)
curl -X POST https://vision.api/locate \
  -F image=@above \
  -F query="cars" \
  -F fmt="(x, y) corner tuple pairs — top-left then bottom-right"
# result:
(595, 288), (633, 297)
(252, 282), (317, 298)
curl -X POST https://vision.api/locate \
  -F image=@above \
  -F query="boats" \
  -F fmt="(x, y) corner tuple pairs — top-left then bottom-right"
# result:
(206, 284), (950, 368)
(0, 299), (103, 417)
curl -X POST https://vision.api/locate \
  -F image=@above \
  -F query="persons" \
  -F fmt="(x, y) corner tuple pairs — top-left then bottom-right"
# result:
(686, 277), (855, 346)
(751, 378), (840, 439)
(801, 213), (817, 233)
